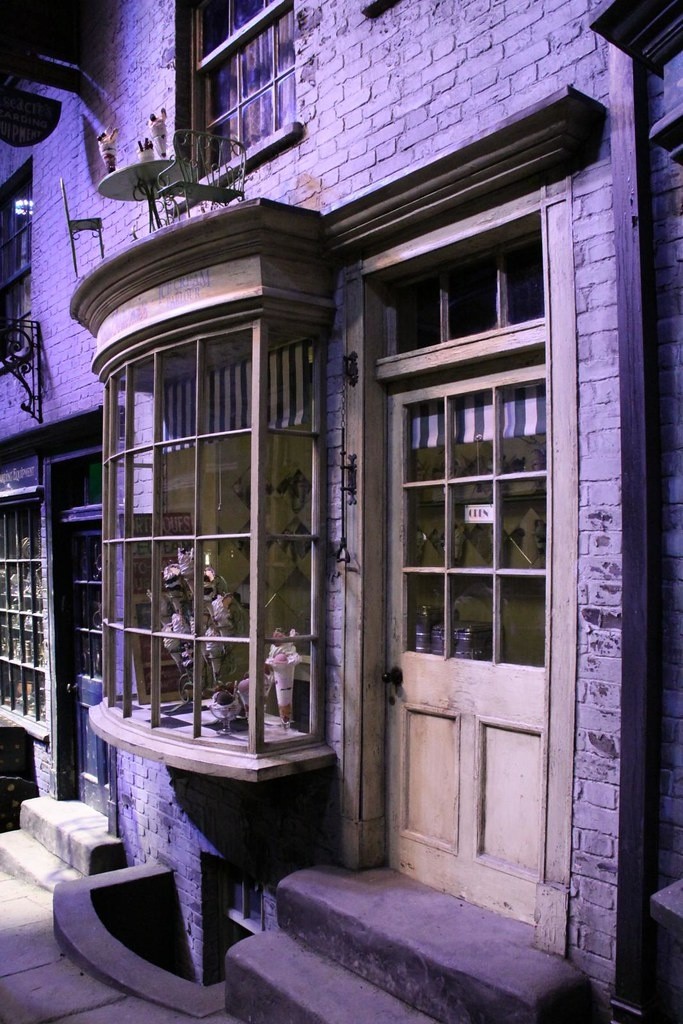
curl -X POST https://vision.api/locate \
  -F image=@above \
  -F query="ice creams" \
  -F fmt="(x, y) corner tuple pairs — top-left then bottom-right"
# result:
(147, 548), (302, 723)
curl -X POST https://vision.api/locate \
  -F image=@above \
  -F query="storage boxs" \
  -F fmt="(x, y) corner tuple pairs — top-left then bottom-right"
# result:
(416, 622), (507, 660)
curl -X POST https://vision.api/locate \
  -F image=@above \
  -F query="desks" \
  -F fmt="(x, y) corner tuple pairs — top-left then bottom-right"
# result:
(98, 159), (195, 234)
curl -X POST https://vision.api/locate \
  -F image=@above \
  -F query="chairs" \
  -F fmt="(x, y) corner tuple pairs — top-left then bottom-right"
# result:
(60, 178), (105, 278)
(134, 131), (246, 237)
